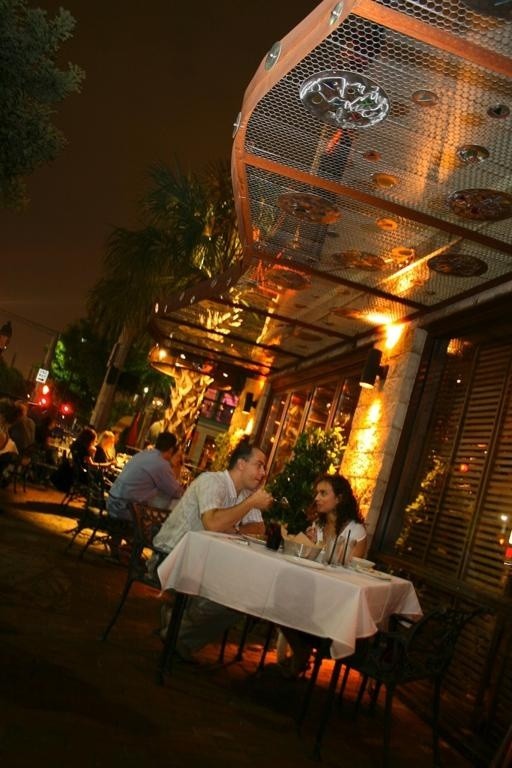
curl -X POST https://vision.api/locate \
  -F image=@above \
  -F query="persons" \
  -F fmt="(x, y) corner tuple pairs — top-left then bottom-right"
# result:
(268, 474), (368, 683)
(1, 396), (121, 510)
(106, 430), (187, 560)
(152, 437), (278, 666)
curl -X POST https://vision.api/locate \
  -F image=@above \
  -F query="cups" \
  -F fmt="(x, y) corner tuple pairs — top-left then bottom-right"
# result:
(323, 535), (353, 566)
(266, 520), (283, 551)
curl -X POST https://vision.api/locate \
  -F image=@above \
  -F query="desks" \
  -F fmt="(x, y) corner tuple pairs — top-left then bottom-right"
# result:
(62, 443), (231, 675)
(157, 527), (412, 733)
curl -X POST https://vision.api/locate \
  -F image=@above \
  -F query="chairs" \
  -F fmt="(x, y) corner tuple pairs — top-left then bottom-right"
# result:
(7, 440), (42, 494)
(297, 596), (484, 767)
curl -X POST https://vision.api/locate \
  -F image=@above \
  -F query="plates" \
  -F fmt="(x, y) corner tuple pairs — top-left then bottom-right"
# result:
(351, 556), (375, 569)
(283, 555), (325, 570)
(356, 567), (392, 581)
(242, 532), (282, 548)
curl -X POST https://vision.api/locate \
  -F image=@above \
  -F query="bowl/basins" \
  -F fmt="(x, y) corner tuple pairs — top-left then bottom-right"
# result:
(284, 537), (322, 560)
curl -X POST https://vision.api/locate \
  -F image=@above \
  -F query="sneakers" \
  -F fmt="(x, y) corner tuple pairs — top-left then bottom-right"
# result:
(278, 654), (311, 676)
(161, 603), (200, 665)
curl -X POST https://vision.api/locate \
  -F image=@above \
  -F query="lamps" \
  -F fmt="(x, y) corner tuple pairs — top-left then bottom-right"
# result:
(242, 392), (257, 414)
(358, 348), (388, 390)
